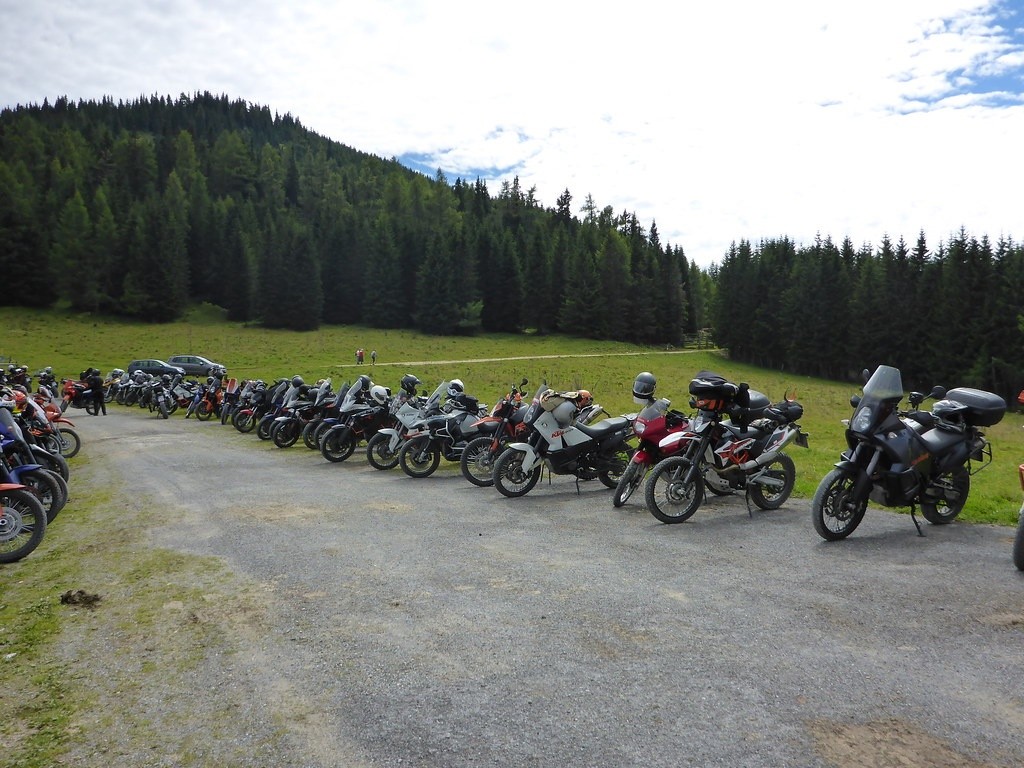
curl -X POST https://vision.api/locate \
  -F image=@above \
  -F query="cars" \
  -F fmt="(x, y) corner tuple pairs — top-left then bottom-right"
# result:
(128, 359), (187, 378)
(168, 354), (228, 376)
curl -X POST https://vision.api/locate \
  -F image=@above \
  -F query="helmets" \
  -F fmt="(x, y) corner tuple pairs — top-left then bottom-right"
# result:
(134, 370), (143, 376)
(113, 369), (119, 375)
(447, 379), (465, 397)
(574, 390), (593, 408)
(279, 378), (290, 386)
(161, 374), (172, 381)
(632, 371), (656, 399)
(255, 380), (263, 390)
(291, 374), (305, 387)
(309, 387), (319, 403)
(0, 365), (53, 376)
(118, 370), (124, 375)
(357, 375), (373, 393)
(552, 400), (576, 430)
(175, 374), (183, 381)
(92, 369), (101, 376)
(371, 384), (387, 404)
(400, 373), (421, 393)
(207, 377), (214, 385)
(145, 374), (153, 380)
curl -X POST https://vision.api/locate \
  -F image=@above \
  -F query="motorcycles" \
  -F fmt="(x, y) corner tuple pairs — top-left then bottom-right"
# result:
(493, 378), (642, 498)
(0, 364), (533, 526)
(612, 372), (746, 507)
(644, 370), (811, 525)
(811, 365), (1008, 543)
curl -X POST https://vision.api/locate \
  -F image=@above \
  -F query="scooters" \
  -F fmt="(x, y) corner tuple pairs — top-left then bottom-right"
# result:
(0, 484), (47, 563)
(1011, 390), (1024, 572)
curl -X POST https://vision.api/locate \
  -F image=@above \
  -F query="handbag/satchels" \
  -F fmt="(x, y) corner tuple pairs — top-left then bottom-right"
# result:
(689, 370), (741, 399)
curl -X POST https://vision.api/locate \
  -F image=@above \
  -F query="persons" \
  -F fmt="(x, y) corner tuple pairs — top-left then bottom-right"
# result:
(87, 369), (107, 416)
(371, 350), (376, 365)
(357, 348), (363, 365)
